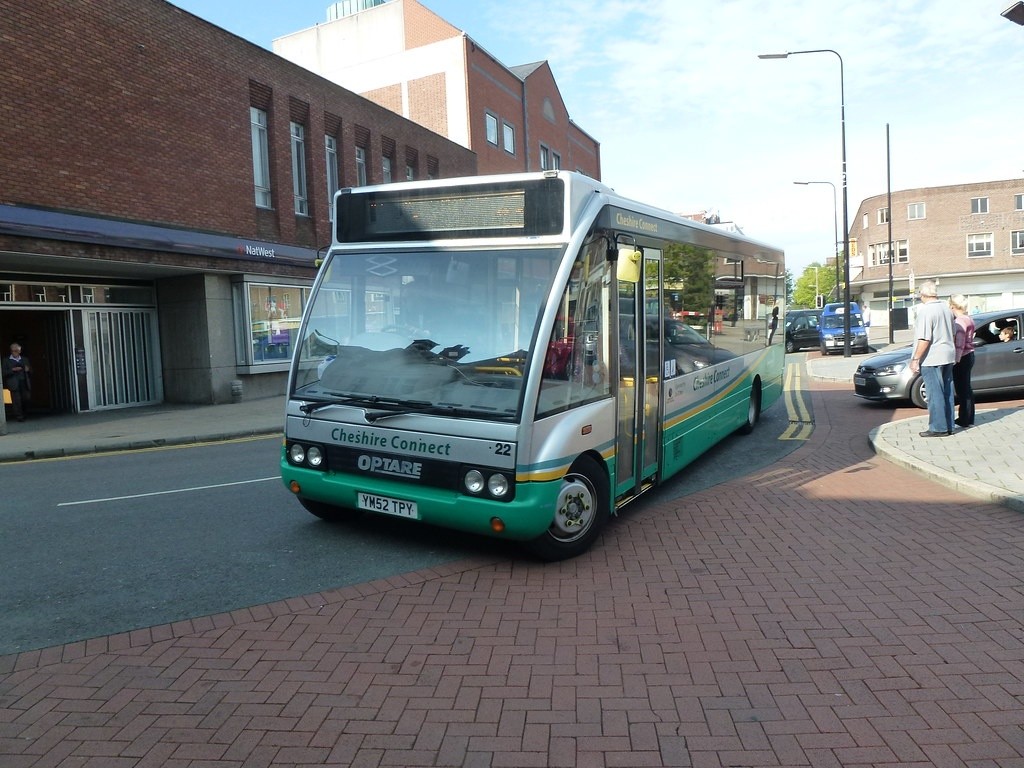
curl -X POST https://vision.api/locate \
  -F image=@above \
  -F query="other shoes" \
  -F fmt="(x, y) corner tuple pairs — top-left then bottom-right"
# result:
(949, 431), (955, 435)
(919, 430), (948, 437)
(954, 418), (969, 427)
(19, 418), (25, 422)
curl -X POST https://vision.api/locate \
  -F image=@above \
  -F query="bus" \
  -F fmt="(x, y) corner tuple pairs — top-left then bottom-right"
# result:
(279, 170), (785, 562)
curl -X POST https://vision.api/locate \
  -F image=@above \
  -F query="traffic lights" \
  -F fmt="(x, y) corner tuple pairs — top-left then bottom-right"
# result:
(817, 296), (823, 308)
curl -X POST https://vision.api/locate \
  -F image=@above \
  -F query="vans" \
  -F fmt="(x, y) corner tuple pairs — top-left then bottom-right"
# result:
(786, 302), (869, 356)
(619, 298), (668, 317)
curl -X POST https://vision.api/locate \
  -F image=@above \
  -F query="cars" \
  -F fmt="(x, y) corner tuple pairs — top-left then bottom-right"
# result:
(853, 308), (1024, 409)
(619, 314), (739, 381)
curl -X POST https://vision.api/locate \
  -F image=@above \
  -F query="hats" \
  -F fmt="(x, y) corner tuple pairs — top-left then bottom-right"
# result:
(919, 282), (937, 296)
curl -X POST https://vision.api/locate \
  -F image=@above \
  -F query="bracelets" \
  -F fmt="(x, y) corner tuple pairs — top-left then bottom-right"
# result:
(912, 357), (919, 360)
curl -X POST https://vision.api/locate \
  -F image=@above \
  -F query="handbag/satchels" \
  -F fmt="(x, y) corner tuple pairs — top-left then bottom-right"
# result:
(25, 368), (41, 393)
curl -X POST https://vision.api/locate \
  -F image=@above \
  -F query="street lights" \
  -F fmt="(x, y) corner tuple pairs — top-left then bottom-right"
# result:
(793, 180), (840, 302)
(758, 49), (851, 358)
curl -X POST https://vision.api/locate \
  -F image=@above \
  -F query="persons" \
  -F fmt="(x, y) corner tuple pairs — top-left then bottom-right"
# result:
(2, 343), (32, 422)
(947, 292), (975, 428)
(999, 326), (1014, 342)
(909, 281), (956, 437)
